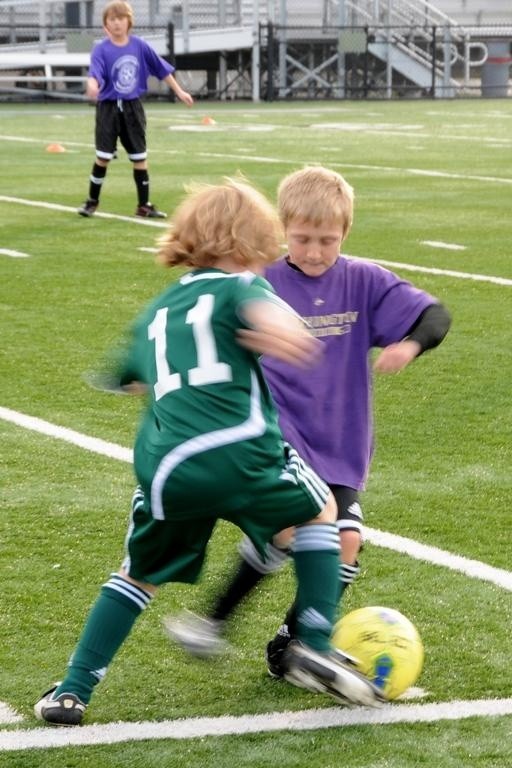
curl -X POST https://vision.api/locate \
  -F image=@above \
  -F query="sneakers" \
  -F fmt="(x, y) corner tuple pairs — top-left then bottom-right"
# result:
(166, 601), (381, 708)
(135, 202), (169, 219)
(79, 197), (99, 220)
(33, 685), (89, 727)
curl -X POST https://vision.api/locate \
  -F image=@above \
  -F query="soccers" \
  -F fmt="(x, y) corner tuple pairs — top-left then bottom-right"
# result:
(327, 607), (424, 697)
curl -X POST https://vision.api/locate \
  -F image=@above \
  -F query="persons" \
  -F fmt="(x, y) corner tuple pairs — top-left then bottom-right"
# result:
(154, 167), (455, 683)
(75, 0), (197, 219)
(33, 167), (378, 727)
